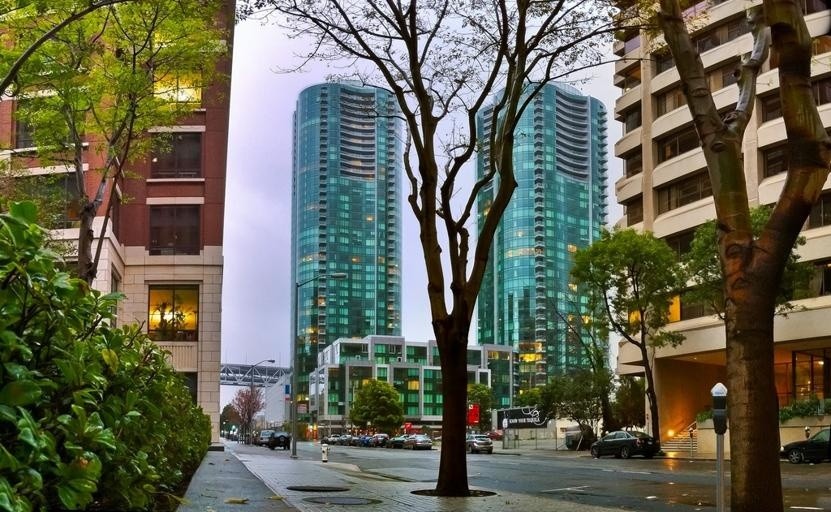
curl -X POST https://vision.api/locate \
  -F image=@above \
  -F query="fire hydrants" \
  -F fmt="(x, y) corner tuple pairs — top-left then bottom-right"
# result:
(319, 443), (330, 464)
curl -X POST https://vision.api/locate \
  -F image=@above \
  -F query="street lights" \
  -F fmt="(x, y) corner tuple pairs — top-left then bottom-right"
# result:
(293, 272), (348, 459)
(249, 358), (275, 445)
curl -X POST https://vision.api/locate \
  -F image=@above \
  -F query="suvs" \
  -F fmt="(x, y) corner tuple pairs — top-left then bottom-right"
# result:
(269, 430), (292, 450)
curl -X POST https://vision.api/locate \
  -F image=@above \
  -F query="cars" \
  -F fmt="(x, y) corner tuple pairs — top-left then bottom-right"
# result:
(466, 433), (493, 455)
(589, 430), (661, 459)
(321, 432), (432, 451)
(256, 429), (273, 446)
